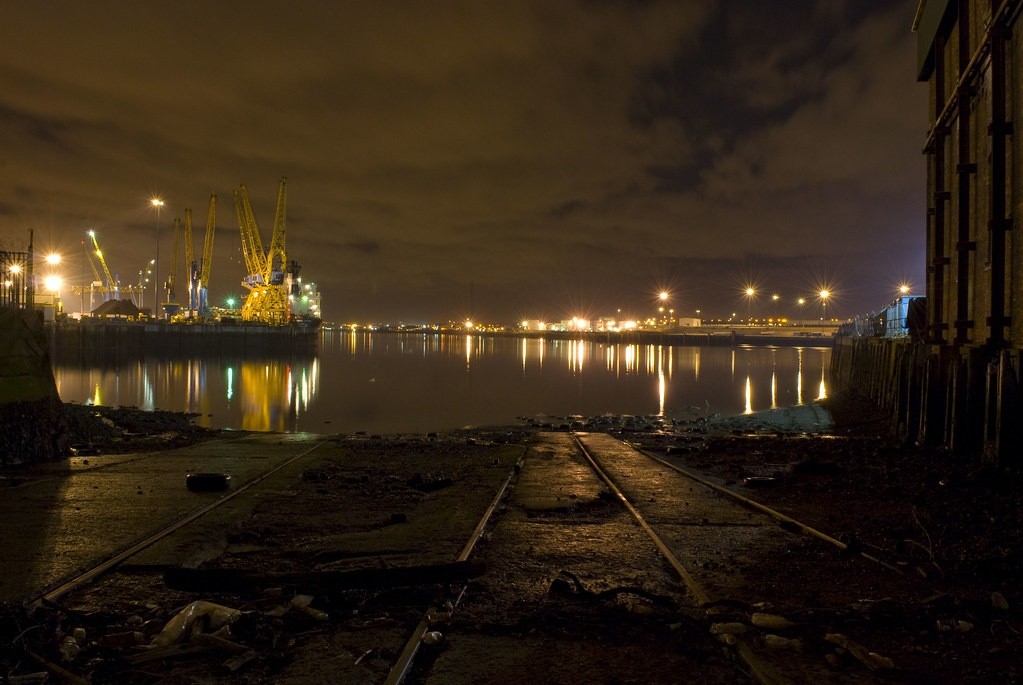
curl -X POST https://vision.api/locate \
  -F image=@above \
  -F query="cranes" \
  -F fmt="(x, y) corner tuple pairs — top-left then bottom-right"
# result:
(167, 187), (220, 325)
(79, 229), (117, 296)
(229, 175), (291, 324)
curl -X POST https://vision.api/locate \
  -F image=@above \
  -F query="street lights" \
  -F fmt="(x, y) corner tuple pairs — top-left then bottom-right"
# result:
(819, 290), (828, 321)
(151, 199), (161, 321)
(81, 230), (95, 317)
(617, 308), (621, 326)
(670, 309), (673, 317)
(747, 288), (753, 320)
